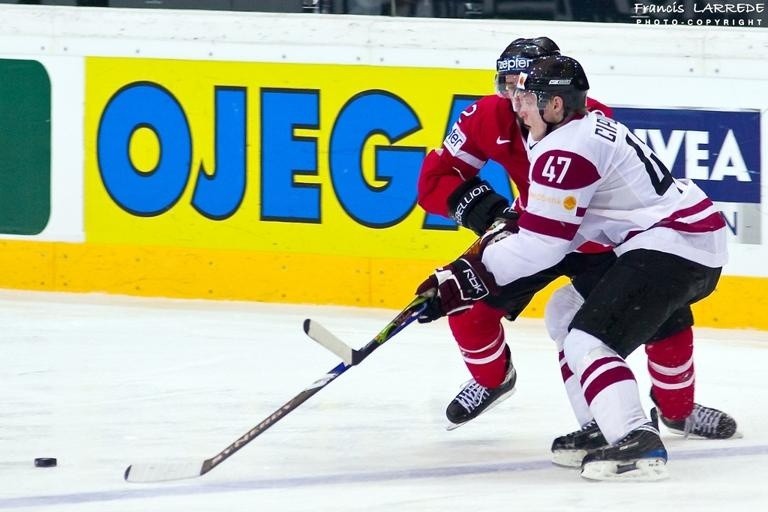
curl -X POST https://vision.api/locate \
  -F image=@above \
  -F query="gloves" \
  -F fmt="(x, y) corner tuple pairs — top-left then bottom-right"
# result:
(447, 173), (520, 239)
(477, 221), (520, 257)
(414, 253), (500, 325)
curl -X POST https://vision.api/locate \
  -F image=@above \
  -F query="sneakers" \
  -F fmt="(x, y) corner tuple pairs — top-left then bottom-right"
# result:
(580, 406), (669, 472)
(648, 400), (737, 440)
(445, 362), (517, 425)
(549, 418), (611, 458)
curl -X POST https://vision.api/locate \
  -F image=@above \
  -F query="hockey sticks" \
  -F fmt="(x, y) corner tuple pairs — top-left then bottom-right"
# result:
(303, 217), (508, 366)
(123, 226), (491, 483)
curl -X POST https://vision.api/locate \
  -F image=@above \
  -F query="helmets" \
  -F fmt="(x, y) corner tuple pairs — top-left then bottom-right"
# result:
(495, 36), (562, 99)
(511, 55), (590, 113)
